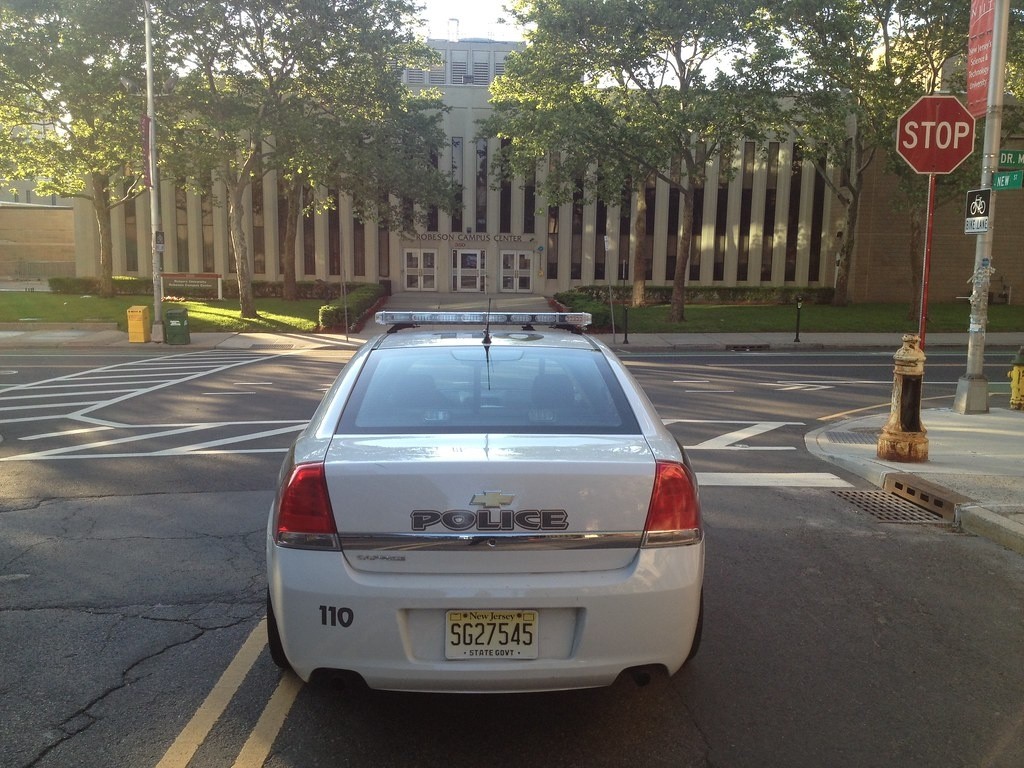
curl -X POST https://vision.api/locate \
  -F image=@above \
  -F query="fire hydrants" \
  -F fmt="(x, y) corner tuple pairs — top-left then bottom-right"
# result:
(1006, 346), (1024, 411)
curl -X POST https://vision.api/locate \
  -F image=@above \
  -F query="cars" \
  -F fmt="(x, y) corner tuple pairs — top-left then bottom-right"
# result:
(263, 310), (707, 696)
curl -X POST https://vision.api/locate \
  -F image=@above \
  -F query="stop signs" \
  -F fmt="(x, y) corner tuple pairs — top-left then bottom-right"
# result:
(896, 96), (975, 175)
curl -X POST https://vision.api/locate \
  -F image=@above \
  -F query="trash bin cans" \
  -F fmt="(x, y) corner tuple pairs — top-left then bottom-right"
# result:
(166, 308), (190, 345)
(127, 305), (150, 342)
